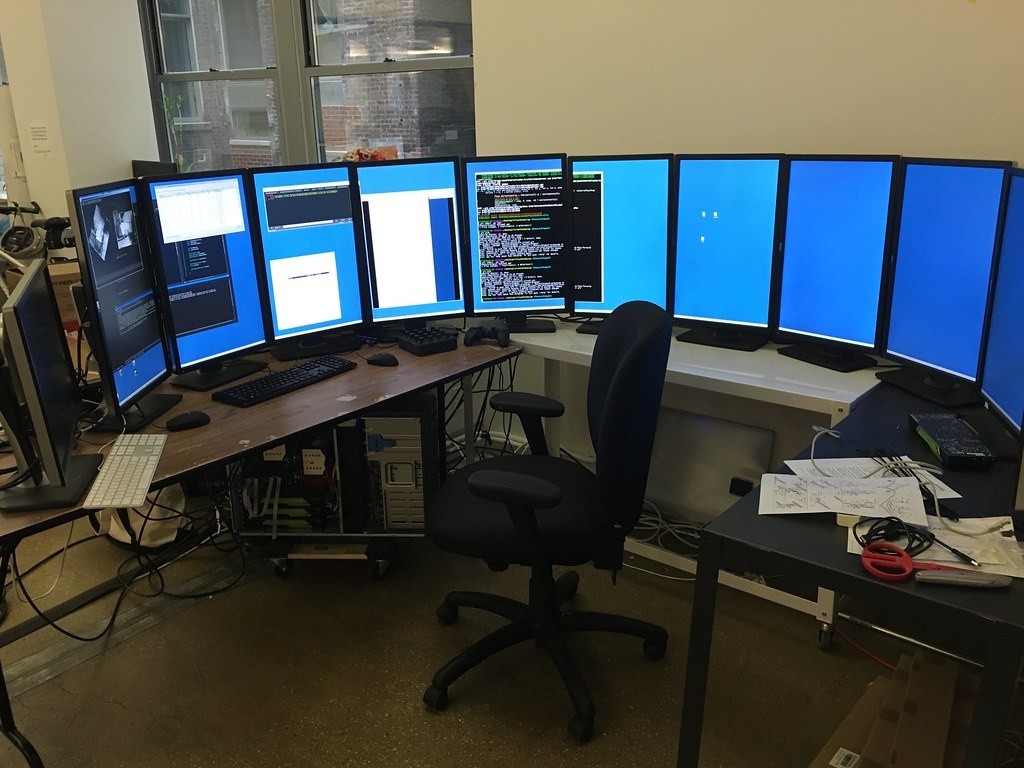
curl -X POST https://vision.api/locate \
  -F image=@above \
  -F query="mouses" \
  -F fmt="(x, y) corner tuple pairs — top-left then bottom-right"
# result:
(165, 411), (211, 432)
(366, 353), (399, 367)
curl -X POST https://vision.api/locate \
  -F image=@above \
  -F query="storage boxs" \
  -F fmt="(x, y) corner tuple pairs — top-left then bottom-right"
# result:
(890, 654), (959, 768)
(861, 653), (912, 768)
(6, 261), (84, 334)
(810, 674), (892, 768)
(942, 667), (980, 768)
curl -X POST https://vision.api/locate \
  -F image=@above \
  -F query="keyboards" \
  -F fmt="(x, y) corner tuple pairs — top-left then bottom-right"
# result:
(83, 433), (169, 510)
(212, 355), (358, 408)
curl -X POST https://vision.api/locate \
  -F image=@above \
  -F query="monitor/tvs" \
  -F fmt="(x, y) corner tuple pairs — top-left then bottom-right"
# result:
(0, 152), (1024, 510)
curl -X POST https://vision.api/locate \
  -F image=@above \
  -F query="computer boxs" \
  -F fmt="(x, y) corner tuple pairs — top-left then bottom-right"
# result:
(224, 394), (440, 538)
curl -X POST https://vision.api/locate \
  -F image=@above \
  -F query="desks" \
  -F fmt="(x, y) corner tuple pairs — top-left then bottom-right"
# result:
(677, 367), (1024, 768)
(0, 329), (523, 768)
(504, 315), (901, 656)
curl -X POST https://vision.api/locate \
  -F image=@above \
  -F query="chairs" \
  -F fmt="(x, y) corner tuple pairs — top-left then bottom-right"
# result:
(421, 299), (673, 746)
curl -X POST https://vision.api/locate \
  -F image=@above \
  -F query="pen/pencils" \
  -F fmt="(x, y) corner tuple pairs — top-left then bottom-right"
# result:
(861, 541), (972, 585)
